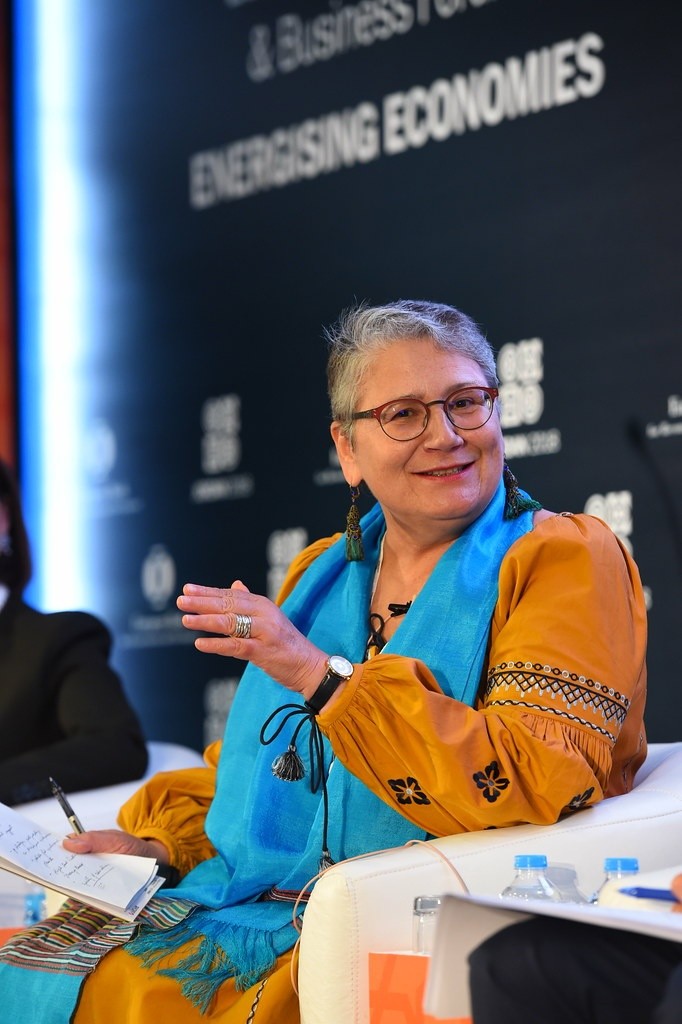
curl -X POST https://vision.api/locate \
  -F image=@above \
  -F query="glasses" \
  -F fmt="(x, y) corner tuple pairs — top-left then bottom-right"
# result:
(336, 386), (498, 441)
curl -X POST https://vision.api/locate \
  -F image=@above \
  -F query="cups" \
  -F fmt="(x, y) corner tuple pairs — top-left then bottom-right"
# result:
(413, 898), (440, 954)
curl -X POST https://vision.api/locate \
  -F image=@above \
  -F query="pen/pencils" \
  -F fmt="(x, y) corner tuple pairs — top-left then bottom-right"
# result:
(48, 776), (86, 836)
(618, 886), (678, 902)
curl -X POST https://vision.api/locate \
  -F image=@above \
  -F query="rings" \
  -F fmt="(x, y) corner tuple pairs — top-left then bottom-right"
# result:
(230, 613), (252, 639)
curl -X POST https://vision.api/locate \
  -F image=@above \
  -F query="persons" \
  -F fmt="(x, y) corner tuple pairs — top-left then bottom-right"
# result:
(0, 456), (149, 806)
(0, 300), (647, 1024)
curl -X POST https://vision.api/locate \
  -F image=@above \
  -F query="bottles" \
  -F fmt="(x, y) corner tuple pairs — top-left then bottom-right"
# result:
(24, 881), (47, 926)
(500, 856), (564, 903)
(590, 859), (639, 905)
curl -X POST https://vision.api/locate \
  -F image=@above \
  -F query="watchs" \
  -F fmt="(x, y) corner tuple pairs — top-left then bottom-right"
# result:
(304, 654), (354, 715)
